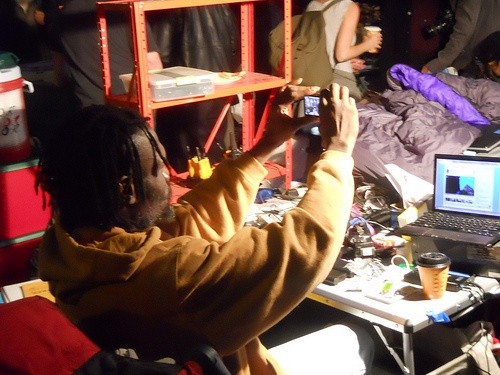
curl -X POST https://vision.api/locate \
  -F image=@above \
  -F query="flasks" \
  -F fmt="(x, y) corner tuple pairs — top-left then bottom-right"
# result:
(0, 53), (34, 166)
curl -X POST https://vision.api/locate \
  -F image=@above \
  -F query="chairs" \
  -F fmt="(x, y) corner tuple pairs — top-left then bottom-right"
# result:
(79, 311), (232, 375)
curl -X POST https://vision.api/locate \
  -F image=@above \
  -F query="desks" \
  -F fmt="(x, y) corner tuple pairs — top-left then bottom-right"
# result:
(262, 226), (500, 375)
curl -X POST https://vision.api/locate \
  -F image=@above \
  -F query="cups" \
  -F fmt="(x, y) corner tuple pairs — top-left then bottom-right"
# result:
(363, 26), (381, 53)
(416, 251), (451, 299)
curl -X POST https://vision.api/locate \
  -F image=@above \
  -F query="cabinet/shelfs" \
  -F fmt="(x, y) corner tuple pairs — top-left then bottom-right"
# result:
(97, 1), (292, 198)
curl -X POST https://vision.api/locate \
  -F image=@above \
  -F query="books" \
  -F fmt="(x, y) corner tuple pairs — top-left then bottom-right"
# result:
(468, 132), (500, 152)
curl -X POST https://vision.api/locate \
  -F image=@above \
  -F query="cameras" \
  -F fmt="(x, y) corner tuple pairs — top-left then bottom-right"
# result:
(304, 95), (320, 117)
(424, 7), (455, 36)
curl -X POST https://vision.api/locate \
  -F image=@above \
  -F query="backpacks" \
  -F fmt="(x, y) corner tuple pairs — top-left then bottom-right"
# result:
(268, 0), (341, 91)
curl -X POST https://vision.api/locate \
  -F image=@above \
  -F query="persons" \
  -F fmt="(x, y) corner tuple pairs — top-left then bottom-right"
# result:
(34, 0), (242, 163)
(305, 0), (383, 92)
(37, 78), (376, 375)
(419, 0), (500, 80)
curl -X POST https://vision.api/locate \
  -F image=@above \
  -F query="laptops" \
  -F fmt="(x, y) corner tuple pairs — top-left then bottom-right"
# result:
(395, 154), (500, 249)
